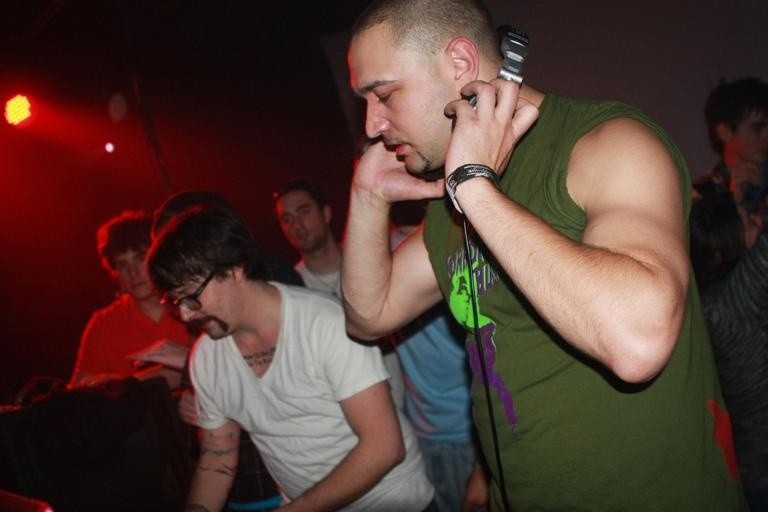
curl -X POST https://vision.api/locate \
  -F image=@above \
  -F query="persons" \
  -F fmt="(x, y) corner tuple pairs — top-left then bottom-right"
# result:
(690, 76), (768, 226)
(339, 1), (748, 512)
(350, 131), (491, 512)
(145, 206), (454, 511)
(692, 191), (768, 512)
(123, 194), (307, 512)
(272, 178), (407, 410)
(68, 209), (196, 396)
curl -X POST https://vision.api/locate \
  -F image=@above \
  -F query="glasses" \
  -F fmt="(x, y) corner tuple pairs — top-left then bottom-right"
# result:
(166, 265), (223, 311)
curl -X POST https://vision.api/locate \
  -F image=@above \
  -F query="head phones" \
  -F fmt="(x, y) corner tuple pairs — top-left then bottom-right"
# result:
(451, 23), (531, 134)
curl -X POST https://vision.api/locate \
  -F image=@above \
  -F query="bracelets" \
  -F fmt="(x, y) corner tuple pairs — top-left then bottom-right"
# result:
(444, 164), (503, 215)
